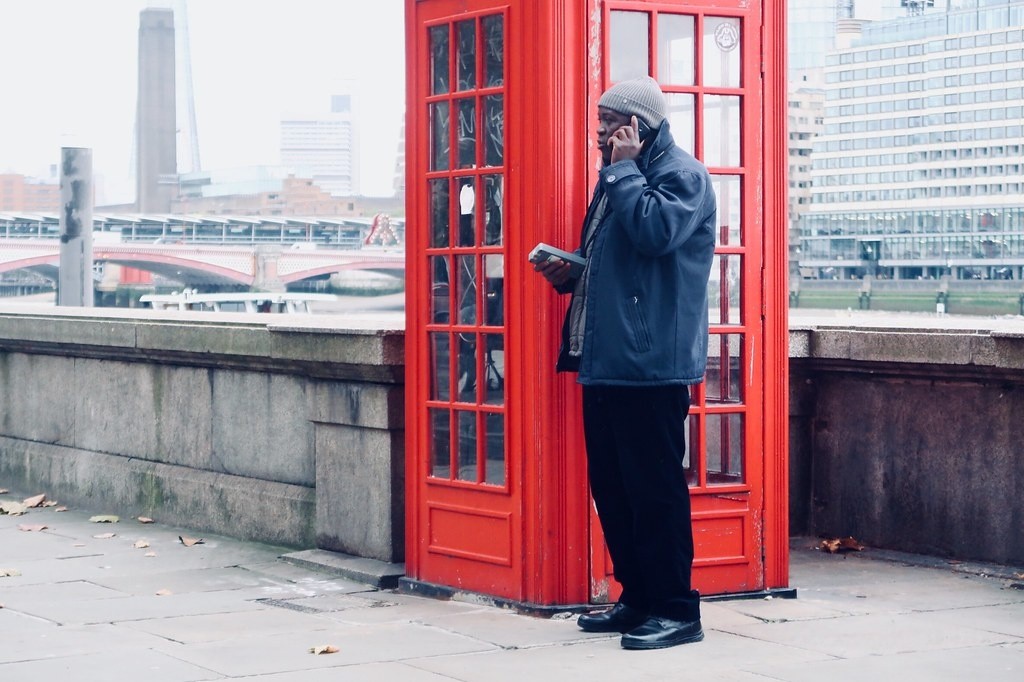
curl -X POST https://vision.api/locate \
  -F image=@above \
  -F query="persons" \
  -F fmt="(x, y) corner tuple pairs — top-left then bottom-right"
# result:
(535, 75), (716, 650)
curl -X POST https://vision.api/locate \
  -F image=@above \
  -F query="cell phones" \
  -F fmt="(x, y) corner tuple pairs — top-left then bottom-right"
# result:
(609, 118), (651, 150)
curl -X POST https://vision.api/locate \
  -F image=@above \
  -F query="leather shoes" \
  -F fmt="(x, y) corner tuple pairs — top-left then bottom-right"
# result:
(577, 601), (705, 649)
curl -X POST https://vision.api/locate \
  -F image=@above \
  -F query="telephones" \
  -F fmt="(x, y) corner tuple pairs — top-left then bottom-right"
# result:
(458, 176), (501, 247)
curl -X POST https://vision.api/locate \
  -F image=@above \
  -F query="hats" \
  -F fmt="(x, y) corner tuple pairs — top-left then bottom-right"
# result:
(597, 76), (667, 130)
